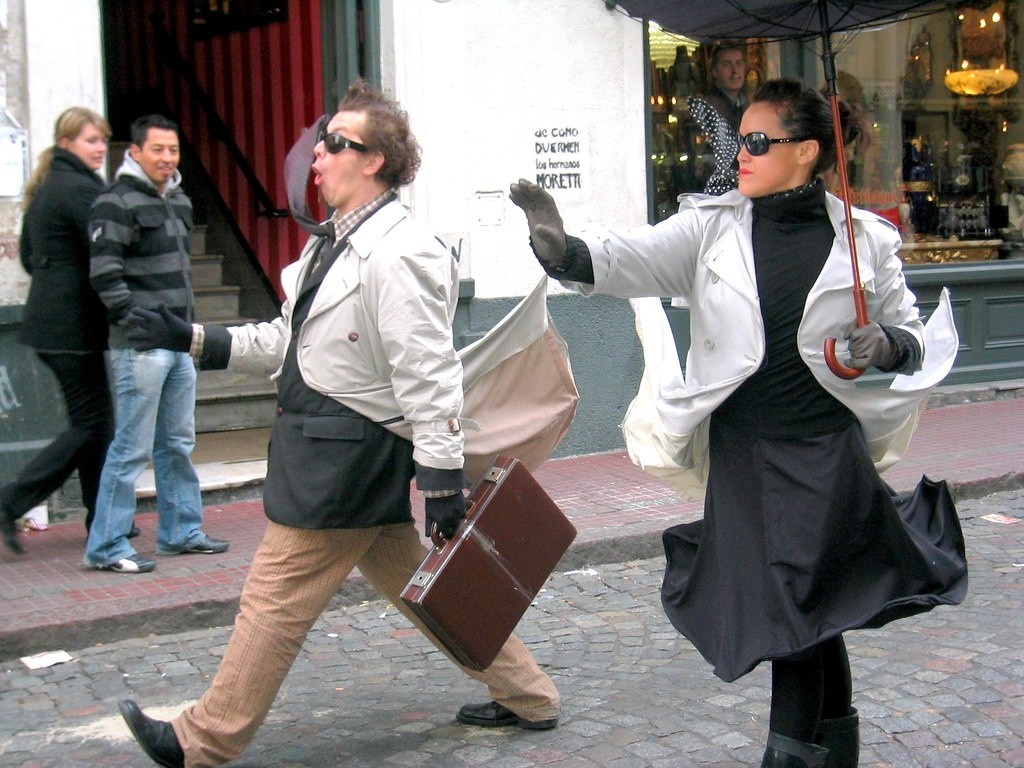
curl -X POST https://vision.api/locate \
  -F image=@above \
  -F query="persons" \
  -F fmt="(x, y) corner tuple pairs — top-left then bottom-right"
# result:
(0, 106), (115, 555)
(821, 70), (878, 219)
(116, 76), (581, 768)
(510, 75), (968, 768)
(85, 114), (232, 573)
(675, 43), (749, 210)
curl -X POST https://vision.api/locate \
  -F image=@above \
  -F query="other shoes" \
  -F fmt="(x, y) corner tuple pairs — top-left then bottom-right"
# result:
(128, 527), (140, 539)
(0, 502), (28, 555)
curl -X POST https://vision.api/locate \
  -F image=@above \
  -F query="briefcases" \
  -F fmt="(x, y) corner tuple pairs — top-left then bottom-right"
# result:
(397, 454), (578, 673)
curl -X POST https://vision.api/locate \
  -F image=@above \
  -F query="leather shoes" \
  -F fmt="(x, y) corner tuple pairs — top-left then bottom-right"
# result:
(456, 700), (559, 729)
(118, 699), (185, 768)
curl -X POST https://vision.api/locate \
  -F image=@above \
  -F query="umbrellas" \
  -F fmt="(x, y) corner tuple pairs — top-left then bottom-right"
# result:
(602, 0), (991, 379)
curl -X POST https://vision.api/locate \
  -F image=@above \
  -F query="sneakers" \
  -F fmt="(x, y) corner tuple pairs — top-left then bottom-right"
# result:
(108, 554), (156, 573)
(185, 534), (230, 555)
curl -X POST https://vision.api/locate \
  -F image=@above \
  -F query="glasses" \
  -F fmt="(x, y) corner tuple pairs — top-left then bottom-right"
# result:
(317, 127), (370, 153)
(736, 131), (804, 156)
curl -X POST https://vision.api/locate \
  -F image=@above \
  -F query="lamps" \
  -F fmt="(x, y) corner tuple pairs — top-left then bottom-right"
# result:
(944, 66), (1020, 97)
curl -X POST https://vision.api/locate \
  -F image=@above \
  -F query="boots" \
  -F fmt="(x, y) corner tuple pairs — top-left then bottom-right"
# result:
(814, 706), (861, 767)
(759, 731), (830, 767)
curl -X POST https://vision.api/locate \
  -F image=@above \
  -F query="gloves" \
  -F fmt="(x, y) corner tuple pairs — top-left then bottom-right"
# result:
(509, 178), (567, 271)
(843, 318), (891, 369)
